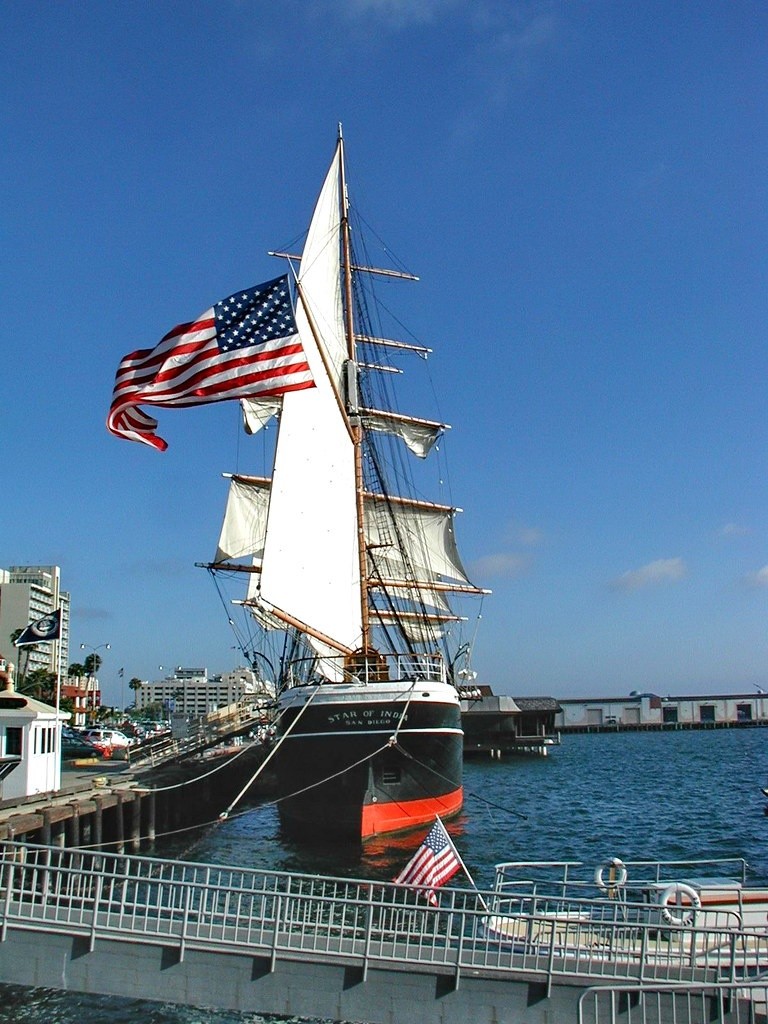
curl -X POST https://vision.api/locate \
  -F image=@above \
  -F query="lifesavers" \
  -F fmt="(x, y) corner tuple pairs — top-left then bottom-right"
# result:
(593, 856), (628, 896)
(658, 882), (702, 927)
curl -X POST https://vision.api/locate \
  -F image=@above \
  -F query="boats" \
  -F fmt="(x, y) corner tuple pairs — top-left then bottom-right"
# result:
(481, 855), (767, 977)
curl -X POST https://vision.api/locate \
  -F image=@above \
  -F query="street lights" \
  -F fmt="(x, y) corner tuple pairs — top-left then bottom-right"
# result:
(80, 643), (111, 723)
(159, 665), (182, 726)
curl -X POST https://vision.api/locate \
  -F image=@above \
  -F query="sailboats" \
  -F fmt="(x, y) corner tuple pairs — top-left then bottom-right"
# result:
(199, 128), (496, 855)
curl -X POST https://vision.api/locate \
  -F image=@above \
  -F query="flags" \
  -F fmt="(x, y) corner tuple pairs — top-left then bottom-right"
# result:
(14, 609), (60, 647)
(390, 820), (461, 907)
(107, 274), (317, 452)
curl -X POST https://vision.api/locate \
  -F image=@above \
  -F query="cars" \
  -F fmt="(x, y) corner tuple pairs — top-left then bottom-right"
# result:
(79, 729), (134, 747)
